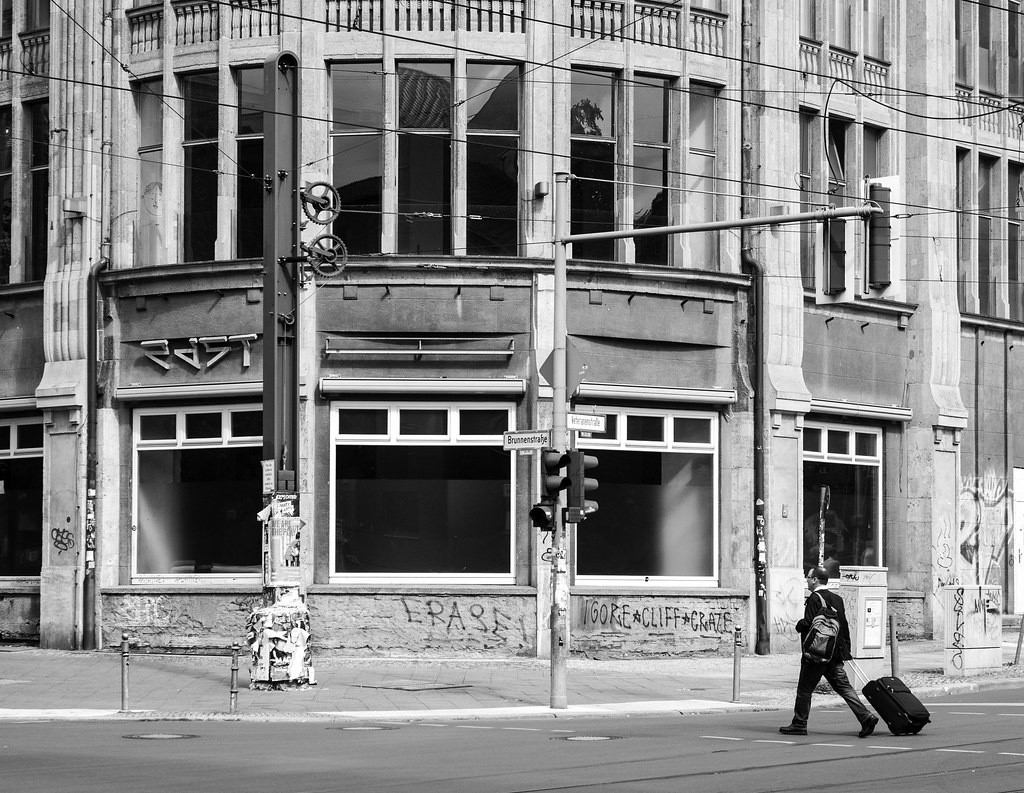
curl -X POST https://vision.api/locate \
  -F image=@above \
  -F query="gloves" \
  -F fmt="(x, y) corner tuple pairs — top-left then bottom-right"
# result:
(841, 652), (853, 661)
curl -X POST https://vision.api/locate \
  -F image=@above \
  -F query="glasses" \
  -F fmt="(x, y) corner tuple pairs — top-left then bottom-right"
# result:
(806, 575), (813, 580)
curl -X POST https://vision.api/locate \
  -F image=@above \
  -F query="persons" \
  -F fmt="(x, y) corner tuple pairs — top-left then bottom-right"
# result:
(777, 566), (879, 739)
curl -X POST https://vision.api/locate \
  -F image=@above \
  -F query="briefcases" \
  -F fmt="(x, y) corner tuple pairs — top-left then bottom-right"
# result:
(847, 660), (931, 736)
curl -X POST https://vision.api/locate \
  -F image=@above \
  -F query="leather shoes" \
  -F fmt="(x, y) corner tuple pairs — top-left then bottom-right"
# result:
(858, 714), (879, 738)
(779, 726), (808, 735)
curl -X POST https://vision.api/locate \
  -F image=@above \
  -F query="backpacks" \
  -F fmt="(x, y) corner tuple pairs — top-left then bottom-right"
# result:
(801, 591), (841, 666)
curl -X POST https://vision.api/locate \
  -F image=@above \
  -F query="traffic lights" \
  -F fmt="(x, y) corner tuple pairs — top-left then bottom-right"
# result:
(536, 445), (572, 503)
(529, 502), (556, 533)
(566, 450), (599, 522)
